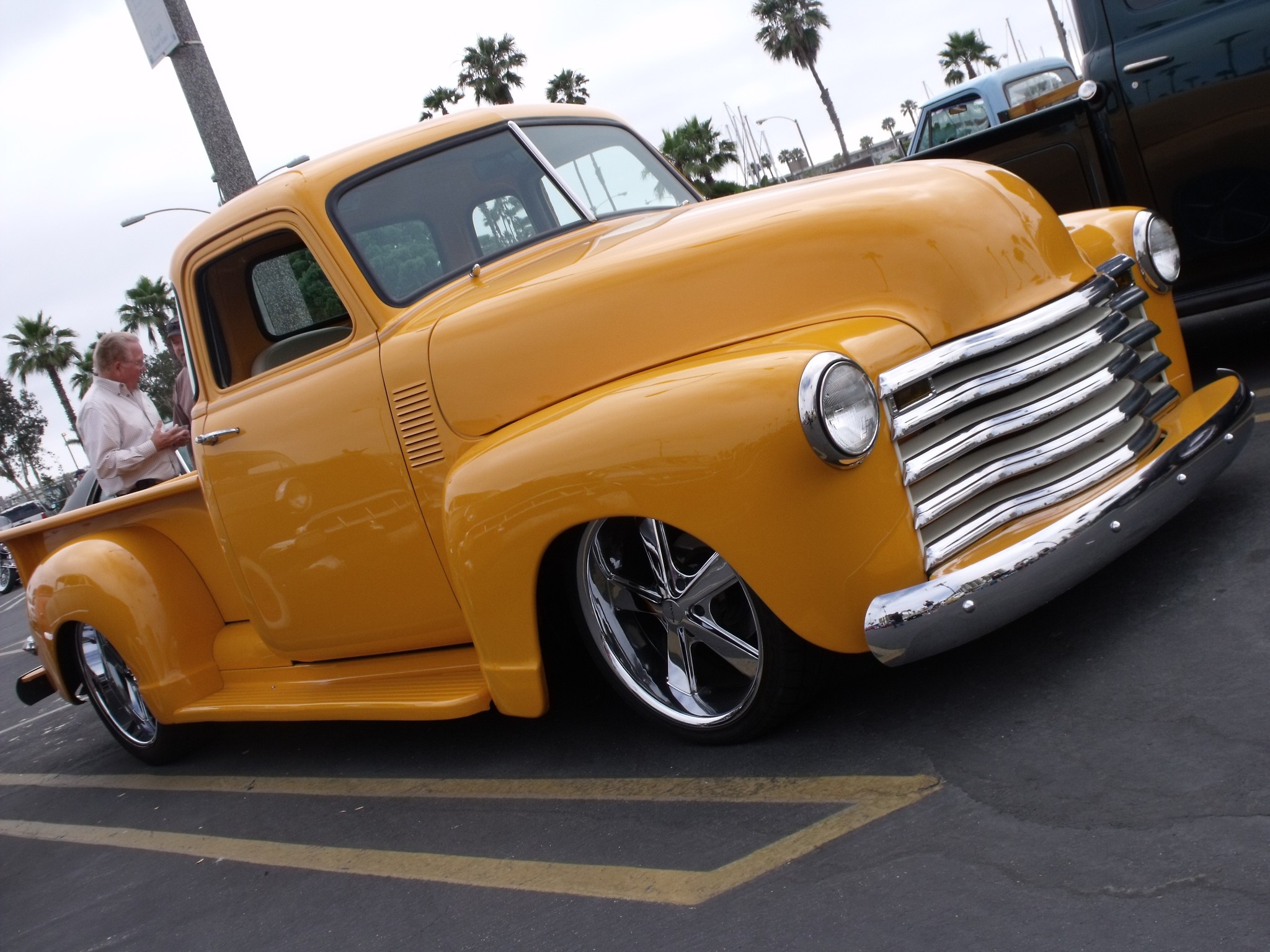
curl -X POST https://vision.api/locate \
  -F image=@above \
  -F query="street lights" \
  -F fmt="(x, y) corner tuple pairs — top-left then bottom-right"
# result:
(753, 116), (818, 172)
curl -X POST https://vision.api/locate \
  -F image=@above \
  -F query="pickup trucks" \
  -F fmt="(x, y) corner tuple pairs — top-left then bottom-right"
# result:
(0, 104), (1255, 761)
(878, 1), (1270, 371)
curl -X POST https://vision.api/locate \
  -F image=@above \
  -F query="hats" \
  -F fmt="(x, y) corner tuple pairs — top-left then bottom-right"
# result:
(73, 469), (85, 478)
(166, 316), (181, 338)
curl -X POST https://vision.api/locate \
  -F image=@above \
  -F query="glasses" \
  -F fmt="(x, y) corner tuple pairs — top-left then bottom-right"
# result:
(113, 360), (144, 369)
(164, 321), (181, 332)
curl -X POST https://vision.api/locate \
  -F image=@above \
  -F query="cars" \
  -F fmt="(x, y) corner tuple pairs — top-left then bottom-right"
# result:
(901, 56), (1075, 155)
(0, 421), (198, 594)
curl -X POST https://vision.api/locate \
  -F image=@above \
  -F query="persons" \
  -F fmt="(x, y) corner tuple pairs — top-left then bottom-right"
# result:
(166, 316), (197, 469)
(74, 468), (85, 481)
(75, 332), (191, 499)
